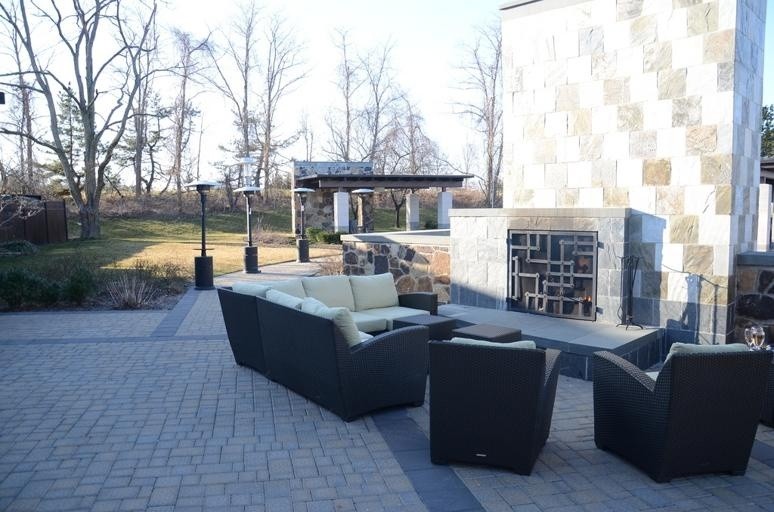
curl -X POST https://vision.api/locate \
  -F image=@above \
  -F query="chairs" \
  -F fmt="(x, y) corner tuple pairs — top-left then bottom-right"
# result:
(426, 338), (560, 476)
(592, 343), (774, 483)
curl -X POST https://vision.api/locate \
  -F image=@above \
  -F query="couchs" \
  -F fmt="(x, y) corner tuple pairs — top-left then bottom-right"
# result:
(216, 272), (438, 422)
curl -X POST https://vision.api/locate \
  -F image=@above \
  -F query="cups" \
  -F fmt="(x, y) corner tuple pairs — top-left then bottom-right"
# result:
(744, 325), (765, 350)
